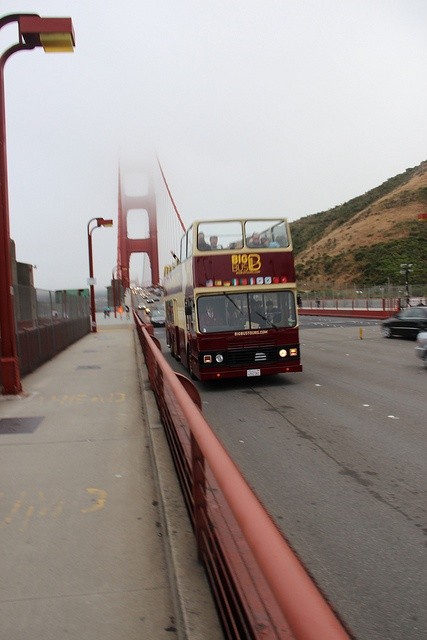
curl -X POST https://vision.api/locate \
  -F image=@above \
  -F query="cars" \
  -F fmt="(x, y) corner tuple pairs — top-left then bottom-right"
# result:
(381, 308), (427, 337)
(151, 311), (165, 327)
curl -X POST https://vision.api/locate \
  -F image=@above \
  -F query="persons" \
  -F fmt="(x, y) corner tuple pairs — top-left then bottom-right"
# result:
(247, 232), (264, 248)
(256, 300), (264, 314)
(198, 232), (211, 251)
(209, 235), (218, 249)
(276, 235), (288, 248)
(104, 305), (131, 320)
(202, 302), (224, 326)
(266, 300), (281, 322)
(261, 238), (270, 248)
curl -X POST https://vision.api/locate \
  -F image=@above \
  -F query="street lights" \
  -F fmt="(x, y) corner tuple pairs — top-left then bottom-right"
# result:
(87, 218), (112, 332)
(118, 267), (127, 309)
(399, 264), (412, 307)
(0, 14), (75, 395)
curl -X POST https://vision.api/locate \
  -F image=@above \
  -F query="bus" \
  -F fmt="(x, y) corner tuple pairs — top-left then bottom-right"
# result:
(163, 218), (302, 380)
